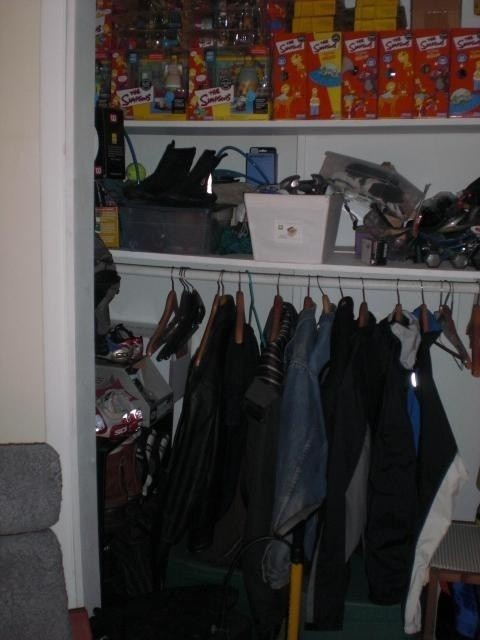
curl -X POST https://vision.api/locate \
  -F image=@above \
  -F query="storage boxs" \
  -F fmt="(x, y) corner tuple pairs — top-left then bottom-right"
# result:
(288, 1), (463, 35)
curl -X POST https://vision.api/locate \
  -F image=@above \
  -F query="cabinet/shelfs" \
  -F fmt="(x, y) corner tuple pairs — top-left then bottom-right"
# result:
(108, 110), (478, 287)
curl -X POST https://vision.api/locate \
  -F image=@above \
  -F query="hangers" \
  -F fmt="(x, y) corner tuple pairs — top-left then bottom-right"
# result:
(133, 259), (478, 383)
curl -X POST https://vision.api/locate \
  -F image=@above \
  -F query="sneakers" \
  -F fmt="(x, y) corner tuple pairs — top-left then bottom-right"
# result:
(96, 323), (142, 365)
(96, 389), (143, 443)
